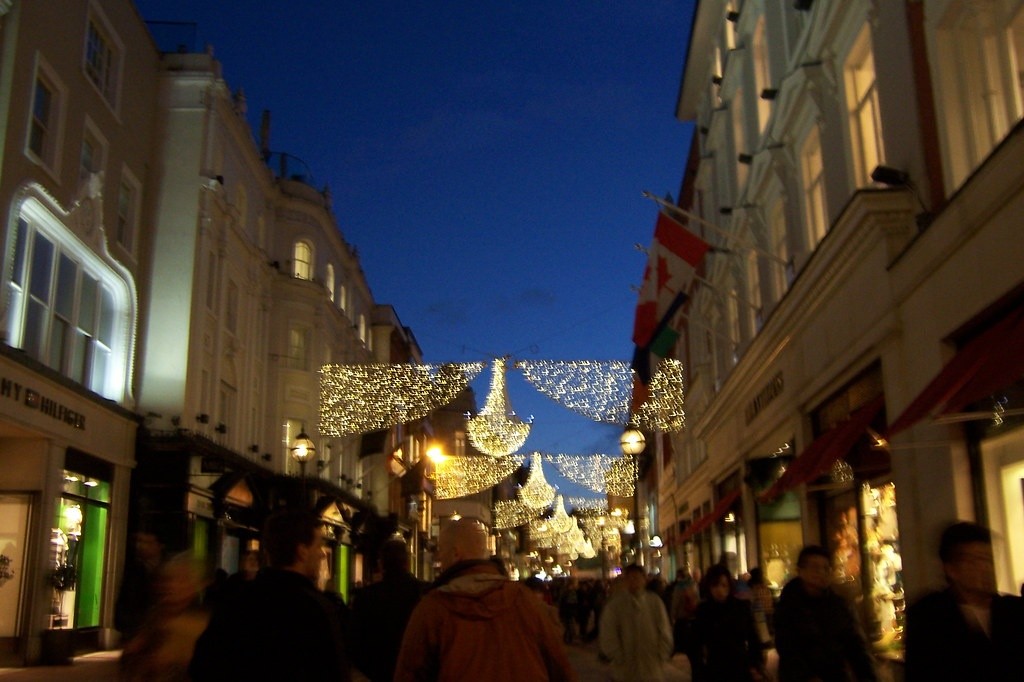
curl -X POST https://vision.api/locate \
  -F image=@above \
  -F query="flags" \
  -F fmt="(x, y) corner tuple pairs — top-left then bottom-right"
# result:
(629, 211), (711, 415)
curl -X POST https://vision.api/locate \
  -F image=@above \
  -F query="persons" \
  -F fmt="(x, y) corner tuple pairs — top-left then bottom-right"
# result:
(526, 575), (610, 644)
(772, 544), (881, 682)
(649, 567), (773, 654)
(351, 539), (434, 682)
(686, 564), (765, 682)
(599, 564), (673, 682)
(394, 519), (580, 682)
(181, 507), (352, 682)
(111, 528), (260, 682)
(901, 522), (1024, 682)
(323, 579), (345, 604)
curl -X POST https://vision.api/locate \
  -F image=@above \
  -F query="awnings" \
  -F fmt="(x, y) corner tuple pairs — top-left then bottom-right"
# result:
(878, 309), (1024, 449)
(675, 492), (741, 546)
(757, 393), (886, 505)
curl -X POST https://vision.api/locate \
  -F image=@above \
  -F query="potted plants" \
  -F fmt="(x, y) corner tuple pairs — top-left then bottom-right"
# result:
(39, 562), (81, 666)
